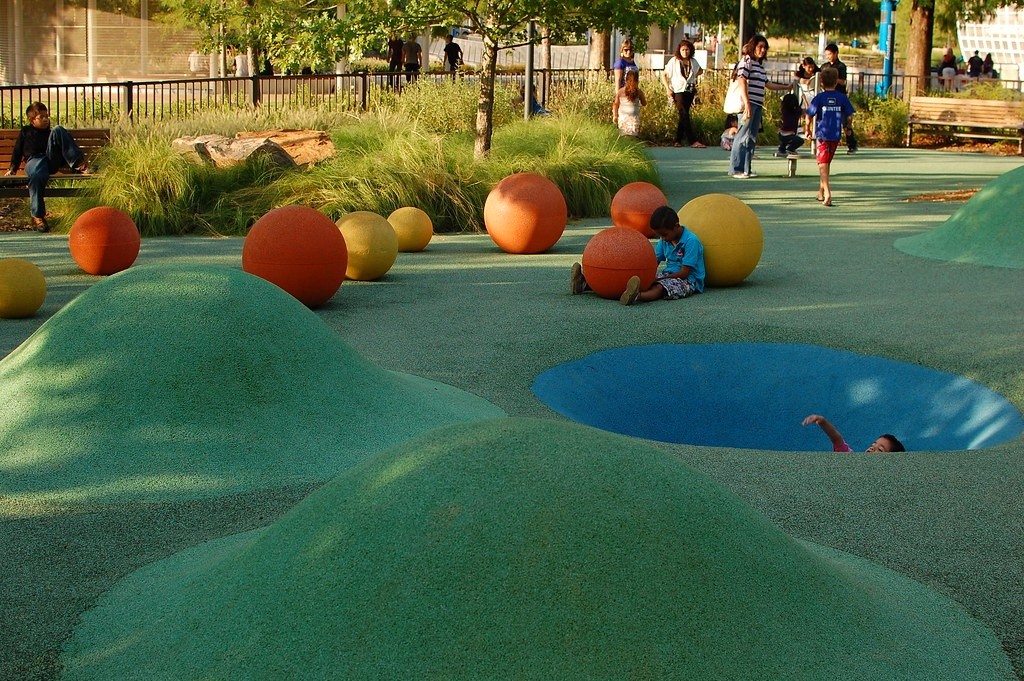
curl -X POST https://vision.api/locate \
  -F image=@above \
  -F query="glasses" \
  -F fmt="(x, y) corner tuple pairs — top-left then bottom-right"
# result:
(624, 48), (633, 51)
(35, 113), (49, 119)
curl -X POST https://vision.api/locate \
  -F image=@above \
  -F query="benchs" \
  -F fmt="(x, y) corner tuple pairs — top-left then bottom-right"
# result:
(907, 96), (1024, 155)
(0, 128), (111, 199)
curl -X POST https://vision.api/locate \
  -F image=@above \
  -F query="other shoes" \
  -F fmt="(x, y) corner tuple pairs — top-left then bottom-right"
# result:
(80, 162), (98, 173)
(33, 216), (49, 232)
(620, 275), (641, 305)
(732, 171), (758, 178)
(571, 261), (587, 294)
(847, 146), (858, 154)
(786, 147), (798, 154)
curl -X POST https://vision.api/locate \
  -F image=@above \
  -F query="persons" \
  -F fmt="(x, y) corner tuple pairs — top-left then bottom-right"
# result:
(520, 83), (547, 114)
(443, 34), (463, 73)
(683, 32), (691, 42)
(938, 48), (958, 86)
(802, 415), (907, 452)
(802, 68), (856, 206)
(819, 43), (858, 154)
(4, 101), (88, 233)
(967, 50), (983, 77)
(613, 71), (647, 136)
(983, 53), (997, 79)
(387, 34), (405, 89)
(571, 206), (706, 306)
(614, 39), (639, 94)
(720, 35), (820, 179)
(662, 40), (707, 148)
(402, 33), (422, 83)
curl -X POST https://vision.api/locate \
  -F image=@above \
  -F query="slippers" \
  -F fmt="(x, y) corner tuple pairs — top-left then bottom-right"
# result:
(674, 141), (682, 146)
(816, 196), (835, 206)
(691, 143), (707, 147)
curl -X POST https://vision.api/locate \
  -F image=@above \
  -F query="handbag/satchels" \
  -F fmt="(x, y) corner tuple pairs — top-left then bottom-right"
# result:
(723, 69), (750, 111)
